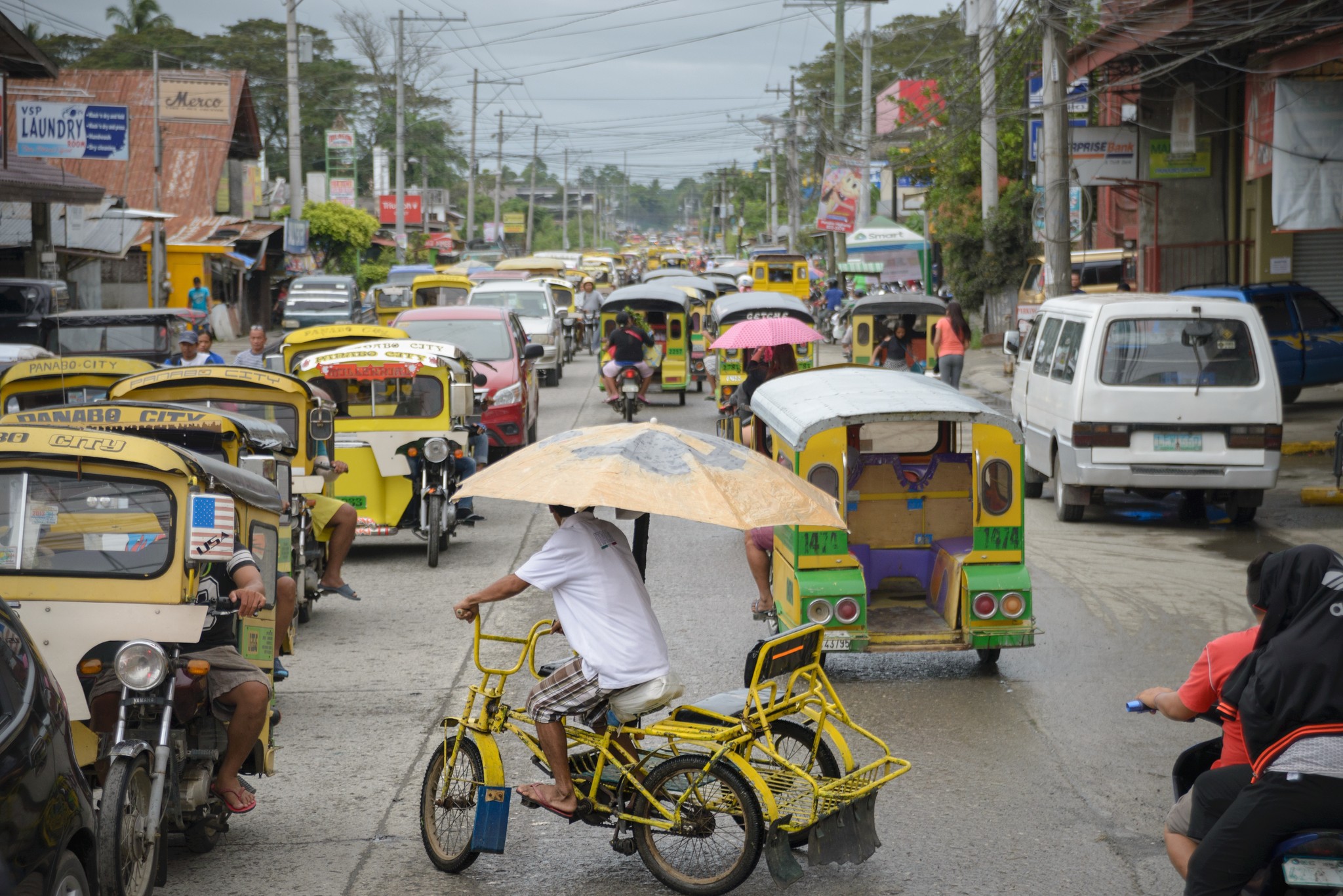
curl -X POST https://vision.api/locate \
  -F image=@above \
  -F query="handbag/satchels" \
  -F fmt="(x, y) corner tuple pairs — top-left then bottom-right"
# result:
(910, 362), (925, 376)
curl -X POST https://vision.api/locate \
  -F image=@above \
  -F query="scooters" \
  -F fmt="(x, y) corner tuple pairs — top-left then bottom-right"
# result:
(1124, 700), (1343, 896)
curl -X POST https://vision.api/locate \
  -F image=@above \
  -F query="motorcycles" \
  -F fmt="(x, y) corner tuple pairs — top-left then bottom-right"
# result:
(749, 361), (1043, 680)
(601, 337), (645, 423)
(0, 277), (497, 896)
(364, 230), (954, 446)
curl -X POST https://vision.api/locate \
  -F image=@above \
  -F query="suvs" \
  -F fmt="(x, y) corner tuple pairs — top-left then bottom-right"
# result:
(1105, 278), (1343, 404)
(468, 280), (567, 387)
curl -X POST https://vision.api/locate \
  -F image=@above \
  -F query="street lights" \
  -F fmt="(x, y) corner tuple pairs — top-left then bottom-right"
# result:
(753, 145), (801, 255)
(466, 171), (503, 250)
(755, 114), (870, 227)
(562, 197), (582, 253)
(758, 167), (794, 253)
(494, 179), (526, 241)
(525, 194), (553, 257)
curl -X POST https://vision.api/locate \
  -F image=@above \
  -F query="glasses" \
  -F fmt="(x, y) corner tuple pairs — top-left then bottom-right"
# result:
(250, 325), (266, 334)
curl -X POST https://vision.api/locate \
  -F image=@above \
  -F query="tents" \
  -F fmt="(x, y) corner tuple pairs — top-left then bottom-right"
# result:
(845, 215), (931, 296)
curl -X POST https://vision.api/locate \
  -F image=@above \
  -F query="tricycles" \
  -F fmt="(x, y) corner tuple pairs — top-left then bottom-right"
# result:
(419, 417), (912, 895)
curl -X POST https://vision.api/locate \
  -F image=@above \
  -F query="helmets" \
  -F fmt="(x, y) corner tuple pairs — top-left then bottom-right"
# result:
(582, 277), (595, 291)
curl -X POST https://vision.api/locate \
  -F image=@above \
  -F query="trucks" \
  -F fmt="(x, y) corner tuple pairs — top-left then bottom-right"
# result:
(277, 278), (376, 336)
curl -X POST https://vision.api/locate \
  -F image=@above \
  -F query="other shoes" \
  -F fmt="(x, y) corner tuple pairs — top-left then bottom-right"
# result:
(605, 395), (619, 403)
(273, 657), (290, 679)
(638, 395), (651, 404)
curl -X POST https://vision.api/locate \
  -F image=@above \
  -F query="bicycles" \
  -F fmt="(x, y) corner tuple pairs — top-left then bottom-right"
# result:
(579, 308), (600, 356)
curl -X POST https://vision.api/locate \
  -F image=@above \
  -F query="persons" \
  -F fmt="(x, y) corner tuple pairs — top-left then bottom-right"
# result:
(87, 492), (275, 813)
(164, 270), (1083, 678)
(452, 503), (671, 813)
(1134, 544), (1343, 896)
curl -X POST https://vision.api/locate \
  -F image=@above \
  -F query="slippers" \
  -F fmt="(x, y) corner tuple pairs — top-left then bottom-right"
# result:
(704, 396), (715, 400)
(317, 585), (361, 600)
(516, 783), (573, 818)
(209, 783), (256, 814)
(750, 600), (773, 614)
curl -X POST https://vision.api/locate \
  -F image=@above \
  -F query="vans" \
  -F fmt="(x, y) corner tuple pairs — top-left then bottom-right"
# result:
(1003, 293), (1282, 529)
(1016, 248), (1143, 356)
(386, 308), (544, 459)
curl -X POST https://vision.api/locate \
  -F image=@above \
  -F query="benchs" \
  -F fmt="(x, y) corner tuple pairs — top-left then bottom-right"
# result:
(668, 623), (823, 738)
(928, 536), (973, 629)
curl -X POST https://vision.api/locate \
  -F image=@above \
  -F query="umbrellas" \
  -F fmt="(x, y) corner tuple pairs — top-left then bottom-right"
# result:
(446, 418), (853, 533)
(708, 318), (825, 365)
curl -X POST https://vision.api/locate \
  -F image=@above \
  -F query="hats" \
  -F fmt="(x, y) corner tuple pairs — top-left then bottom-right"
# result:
(178, 330), (199, 345)
